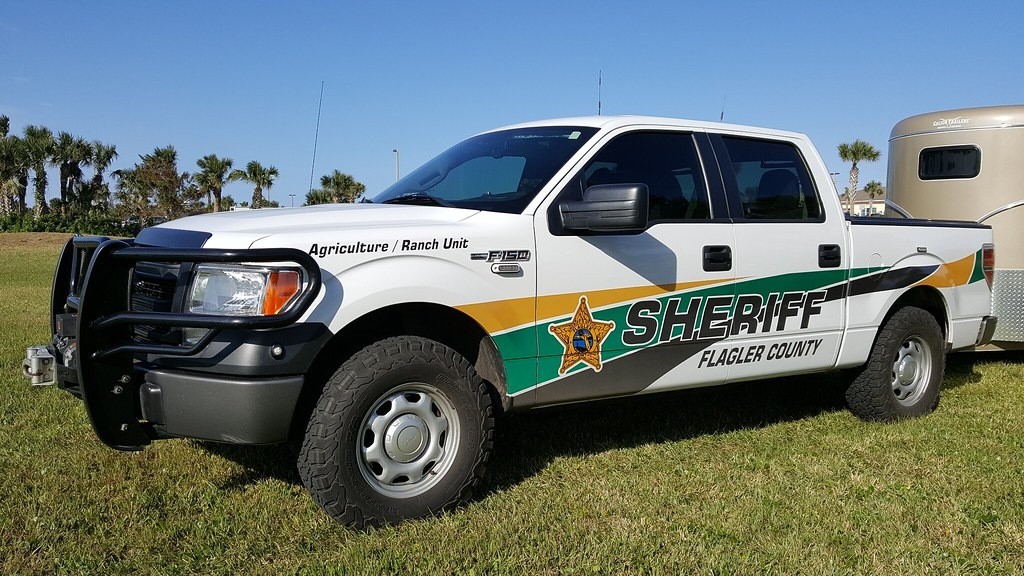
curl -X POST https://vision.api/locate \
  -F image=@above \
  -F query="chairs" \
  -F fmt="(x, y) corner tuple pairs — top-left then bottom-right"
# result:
(584, 166), (613, 187)
(752, 169), (802, 220)
(633, 168), (687, 221)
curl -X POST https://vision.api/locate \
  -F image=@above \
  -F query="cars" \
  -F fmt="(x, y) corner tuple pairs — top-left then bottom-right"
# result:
(120, 214), (170, 231)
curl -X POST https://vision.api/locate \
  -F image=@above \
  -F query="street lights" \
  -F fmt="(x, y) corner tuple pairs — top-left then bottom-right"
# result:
(392, 149), (399, 184)
(830, 172), (840, 186)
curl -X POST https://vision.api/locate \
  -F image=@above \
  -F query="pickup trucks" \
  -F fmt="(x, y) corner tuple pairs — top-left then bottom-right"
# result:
(27, 115), (998, 532)
